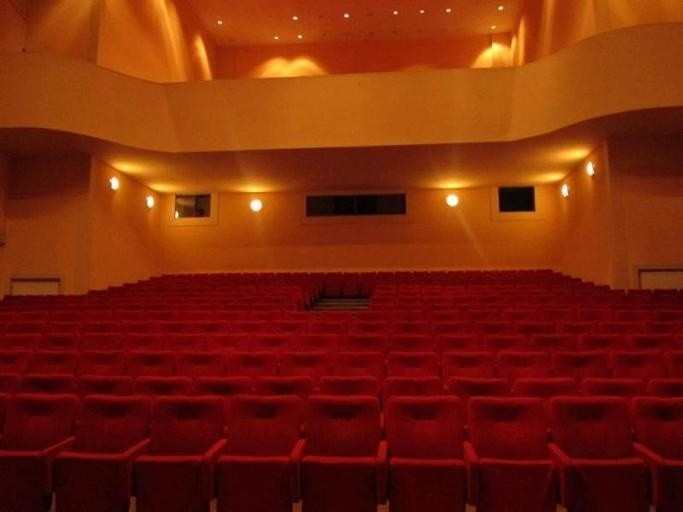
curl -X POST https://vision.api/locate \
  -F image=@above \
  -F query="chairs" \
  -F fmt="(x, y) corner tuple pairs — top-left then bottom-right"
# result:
(0, 268), (683, 511)
